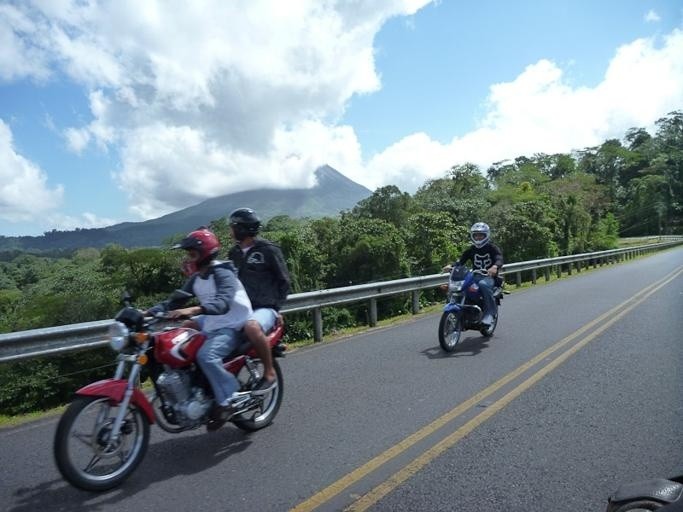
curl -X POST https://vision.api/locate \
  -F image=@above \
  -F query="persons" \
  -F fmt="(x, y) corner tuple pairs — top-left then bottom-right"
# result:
(440, 222), (504, 326)
(140, 228), (254, 432)
(227, 206), (292, 397)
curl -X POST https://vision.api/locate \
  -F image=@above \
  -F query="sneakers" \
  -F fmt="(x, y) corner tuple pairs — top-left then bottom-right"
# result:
(480, 314), (494, 327)
(250, 375), (278, 395)
(206, 402), (234, 432)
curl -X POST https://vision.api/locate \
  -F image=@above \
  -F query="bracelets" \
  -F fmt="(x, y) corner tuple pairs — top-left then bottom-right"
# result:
(492, 264), (498, 269)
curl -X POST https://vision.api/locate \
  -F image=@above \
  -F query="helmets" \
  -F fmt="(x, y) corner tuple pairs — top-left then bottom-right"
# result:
(469, 222), (490, 249)
(180, 226), (222, 278)
(225, 208), (263, 237)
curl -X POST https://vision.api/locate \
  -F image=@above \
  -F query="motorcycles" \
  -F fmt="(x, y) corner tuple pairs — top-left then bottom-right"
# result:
(54, 276), (292, 488)
(437, 258), (504, 353)
